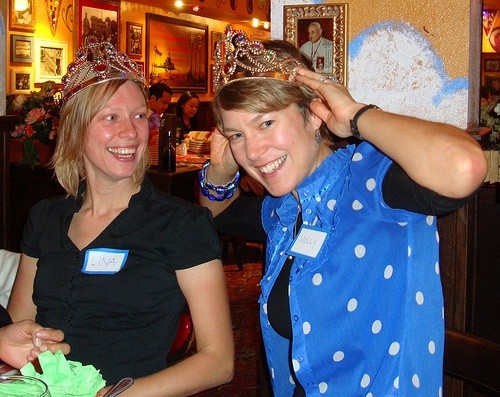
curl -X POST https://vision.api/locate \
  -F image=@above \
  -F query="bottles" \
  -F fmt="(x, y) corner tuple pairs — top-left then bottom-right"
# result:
(157, 118), (176, 172)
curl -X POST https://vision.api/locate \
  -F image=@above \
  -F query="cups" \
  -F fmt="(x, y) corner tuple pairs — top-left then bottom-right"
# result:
(0, 373), (52, 397)
(180, 141), (189, 155)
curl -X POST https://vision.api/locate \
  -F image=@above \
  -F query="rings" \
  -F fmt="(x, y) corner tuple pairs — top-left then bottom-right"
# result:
(321, 74), (338, 81)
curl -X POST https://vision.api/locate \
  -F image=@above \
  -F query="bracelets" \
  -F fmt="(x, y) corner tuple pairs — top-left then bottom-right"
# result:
(350, 104), (381, 139)
(199, 160), (240, 202)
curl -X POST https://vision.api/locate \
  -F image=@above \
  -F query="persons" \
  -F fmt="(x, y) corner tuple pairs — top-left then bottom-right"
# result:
(147, 83), (172, 114)
(194, 29), (487, 397)
(0, 304), (71, 371)
(174, 91), (199, 134)
(298, 22), (332, 73)
(0, 37), (235, 397)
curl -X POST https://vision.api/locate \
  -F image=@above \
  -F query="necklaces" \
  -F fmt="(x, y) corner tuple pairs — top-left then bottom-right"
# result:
(311, 39), (322, 58)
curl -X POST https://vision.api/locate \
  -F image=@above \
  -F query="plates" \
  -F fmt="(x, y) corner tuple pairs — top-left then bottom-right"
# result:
(146, 144), (158, 166)
(189, 139), (212, 154)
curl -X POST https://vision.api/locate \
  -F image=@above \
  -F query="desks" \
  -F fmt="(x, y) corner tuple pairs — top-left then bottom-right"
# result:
(146, 154), (211, 202)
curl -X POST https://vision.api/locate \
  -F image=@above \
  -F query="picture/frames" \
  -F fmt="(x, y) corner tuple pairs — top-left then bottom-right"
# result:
(9, 0), (224, 96)
(284, 3), (348, 88)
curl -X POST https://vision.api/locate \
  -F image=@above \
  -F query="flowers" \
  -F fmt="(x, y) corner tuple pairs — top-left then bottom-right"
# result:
(12, 92), (62, 167)
